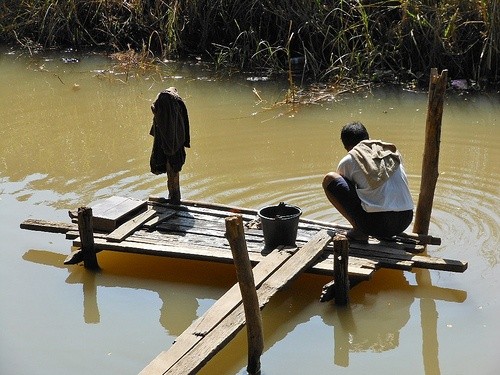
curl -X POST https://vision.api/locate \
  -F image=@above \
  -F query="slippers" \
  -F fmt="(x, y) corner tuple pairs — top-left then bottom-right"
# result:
(344, 230), (368, 245)
(376, 235), (397, 242)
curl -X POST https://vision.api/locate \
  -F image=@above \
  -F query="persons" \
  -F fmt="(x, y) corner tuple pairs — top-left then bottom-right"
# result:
(322, 121), (414, 244)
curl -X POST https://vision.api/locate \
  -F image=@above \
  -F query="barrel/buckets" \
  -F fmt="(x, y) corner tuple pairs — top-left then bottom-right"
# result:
(258, 202), (302, 249)
